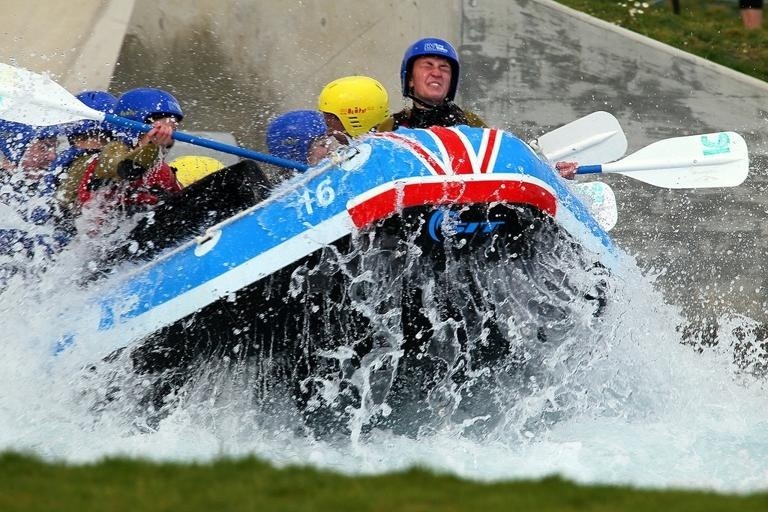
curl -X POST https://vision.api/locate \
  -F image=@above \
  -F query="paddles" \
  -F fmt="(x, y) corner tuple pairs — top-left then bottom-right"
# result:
(527, 111), (749, 233)
(0, 63), (309, 174)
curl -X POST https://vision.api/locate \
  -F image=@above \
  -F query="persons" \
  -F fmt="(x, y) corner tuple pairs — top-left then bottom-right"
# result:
(0, 120), (71, 277)
(265, 108), (337, 185)
(77, 87), (183, 237)
(378, 37), (579, 180)
(31, 90), (118, 251)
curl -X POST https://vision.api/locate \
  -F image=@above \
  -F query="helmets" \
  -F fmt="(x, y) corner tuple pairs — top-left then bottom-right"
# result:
(112, 87), (186, 151)
(399, 38), (461, 102)
(167, 154), (226, 194)
(266, 108), (328, 168)
(0, 90), (120, 193)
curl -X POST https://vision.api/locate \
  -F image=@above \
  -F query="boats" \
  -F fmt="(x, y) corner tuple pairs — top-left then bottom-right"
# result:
(28, 124), (613, 431)
